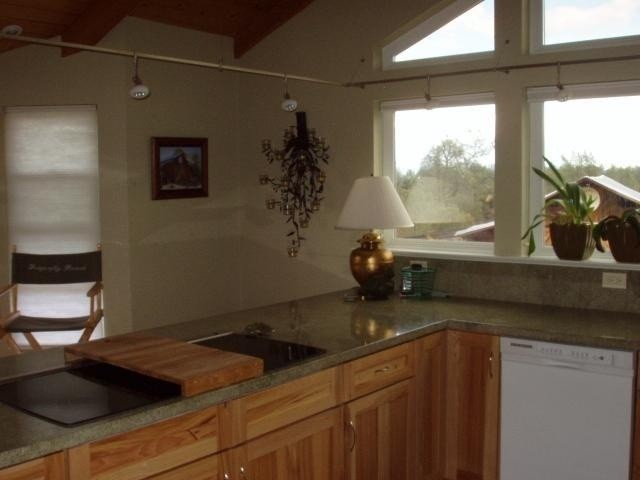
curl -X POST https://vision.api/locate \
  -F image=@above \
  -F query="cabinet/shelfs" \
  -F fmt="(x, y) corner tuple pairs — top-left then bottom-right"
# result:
(231, 328), (444, 480)
(1, 400), (231, 480)
(444, 329), (500, 480)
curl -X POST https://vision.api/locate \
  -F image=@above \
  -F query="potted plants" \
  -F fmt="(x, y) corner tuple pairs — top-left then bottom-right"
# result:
(521, 152), (600, 261)
(592, 205), (640, 264)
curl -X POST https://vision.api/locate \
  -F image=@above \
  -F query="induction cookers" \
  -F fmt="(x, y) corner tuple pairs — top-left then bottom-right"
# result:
(0, 331), (326, 426)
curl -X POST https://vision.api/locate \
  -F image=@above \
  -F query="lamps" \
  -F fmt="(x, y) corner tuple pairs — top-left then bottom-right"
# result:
(280, 72), (298, 113)
(129, 48), (150, 99)
(552, 60), (569, 102)
(334, 173), (415, 303)
(421, 73), (437, 110)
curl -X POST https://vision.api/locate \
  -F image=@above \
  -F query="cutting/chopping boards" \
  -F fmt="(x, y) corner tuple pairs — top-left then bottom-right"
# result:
(65, 330), (265, 398)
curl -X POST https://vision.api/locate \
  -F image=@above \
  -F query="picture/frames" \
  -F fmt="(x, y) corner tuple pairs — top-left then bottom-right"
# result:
(151, 136), (208, 199)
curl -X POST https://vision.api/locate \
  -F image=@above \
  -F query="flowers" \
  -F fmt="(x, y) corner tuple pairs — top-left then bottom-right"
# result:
(255, 110), (331, 259)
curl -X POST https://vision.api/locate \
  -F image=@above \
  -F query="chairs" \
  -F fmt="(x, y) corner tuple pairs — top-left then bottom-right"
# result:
(0, 243), (103, 357)
